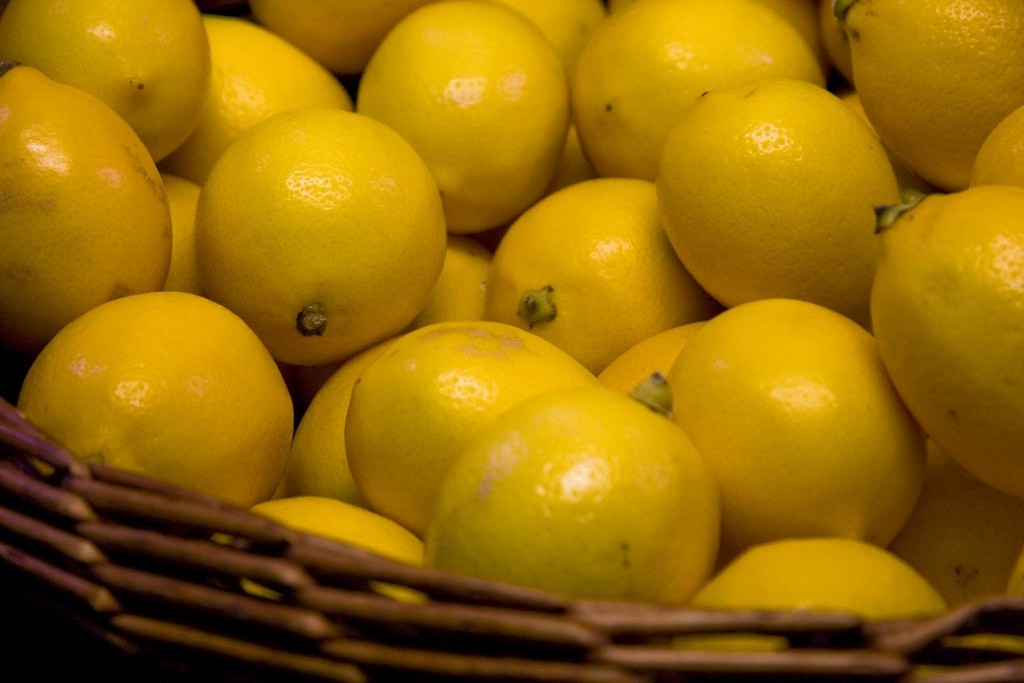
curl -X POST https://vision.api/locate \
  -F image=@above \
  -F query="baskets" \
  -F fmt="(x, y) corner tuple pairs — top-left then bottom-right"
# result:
(0, 403), (1024, 683)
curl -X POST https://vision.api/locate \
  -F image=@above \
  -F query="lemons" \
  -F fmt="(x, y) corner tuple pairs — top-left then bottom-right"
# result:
(0, 0), (1024, 657)
